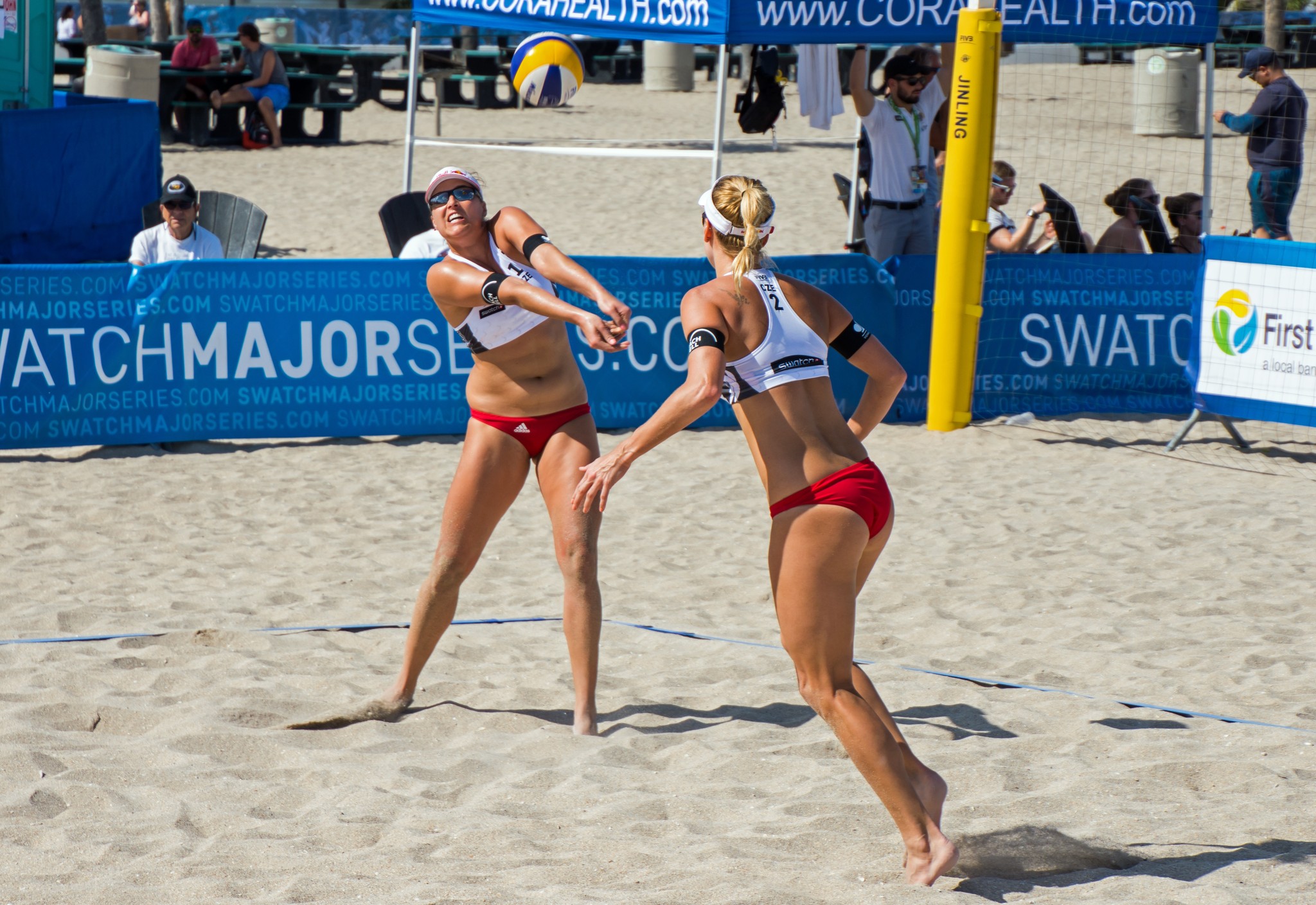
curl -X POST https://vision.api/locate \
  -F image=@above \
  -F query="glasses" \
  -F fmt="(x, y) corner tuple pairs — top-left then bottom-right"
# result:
(428, 186), (483, 212)
(932, 67), (940, 74)
(1248, 66), (1268, 80)
(163, 200), (195, 210)
(992, 181), (1017, 194)
(188, 28), (202, 33)
(1188, 209), (1213, 220)
(895, 77), (927, 87)
(1141, 193), (1162, 206)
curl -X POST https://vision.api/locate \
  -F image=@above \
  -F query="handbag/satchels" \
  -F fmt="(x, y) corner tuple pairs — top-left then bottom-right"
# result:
(738, 44), (785, 135)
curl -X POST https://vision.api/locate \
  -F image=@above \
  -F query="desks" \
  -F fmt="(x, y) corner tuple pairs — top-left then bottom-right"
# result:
(422, 44), (501, 74)
(273, 43), (408, 72)
(156, 67), (336, 101)
(57, 39), (178, 63)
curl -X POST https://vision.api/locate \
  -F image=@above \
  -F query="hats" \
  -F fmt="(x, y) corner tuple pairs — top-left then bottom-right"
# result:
(1237, 47), (1277, 78)
(425, 166), (482, 204)
(160, 174), (196, 204)
(885, 55), (931, 76)
(186, 18), (203, 27)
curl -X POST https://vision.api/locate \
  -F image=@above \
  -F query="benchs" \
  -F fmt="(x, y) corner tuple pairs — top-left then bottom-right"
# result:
(51, 36), (523, 145)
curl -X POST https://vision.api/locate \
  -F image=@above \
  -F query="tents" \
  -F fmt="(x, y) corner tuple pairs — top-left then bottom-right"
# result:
(403, 0), (1215, 245)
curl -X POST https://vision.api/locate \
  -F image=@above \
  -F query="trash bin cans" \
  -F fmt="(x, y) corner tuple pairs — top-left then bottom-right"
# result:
(641, 39), (694, 94)
(1131, 50), (1198, 136)
(253, 17), (295, 42)
(86, 44), (160, 101)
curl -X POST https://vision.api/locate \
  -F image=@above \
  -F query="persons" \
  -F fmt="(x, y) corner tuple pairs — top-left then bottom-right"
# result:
(986, 160), (1291, 257)
(171, 17), (290, 149)
(55, 2), (83, 41)
(849, 39), (954, 268)
(127, 0), (150, 41)
(128, 176), (225, 266)
(571, 174), (964, 886)
(1215, 45), (1310, 238)
(385, 167), (632, 737)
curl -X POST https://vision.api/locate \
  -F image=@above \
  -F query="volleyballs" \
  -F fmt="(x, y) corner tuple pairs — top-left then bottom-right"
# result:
(509, 31), (586, 106)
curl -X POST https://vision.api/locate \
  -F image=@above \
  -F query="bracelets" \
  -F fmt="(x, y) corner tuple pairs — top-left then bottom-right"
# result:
(852, 45), (867, 51)
(831, 318), (871, 359)
(685, 326), (728, 354)
(522, 233), (551, 262)
(482, 270), (508, 304)
(1024, 209), (1040, 220)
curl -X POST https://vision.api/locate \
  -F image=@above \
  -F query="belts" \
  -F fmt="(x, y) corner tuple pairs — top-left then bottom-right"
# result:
(870, 196), (926, 212)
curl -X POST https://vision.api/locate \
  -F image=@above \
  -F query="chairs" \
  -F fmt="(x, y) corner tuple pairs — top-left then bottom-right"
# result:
(140, 191), (268, 258)
(1131, 194), (1175, 254)
(834, 172), (873, 256)
(1039, 183), (1089, 253)
(379, 191), (437, 259)
